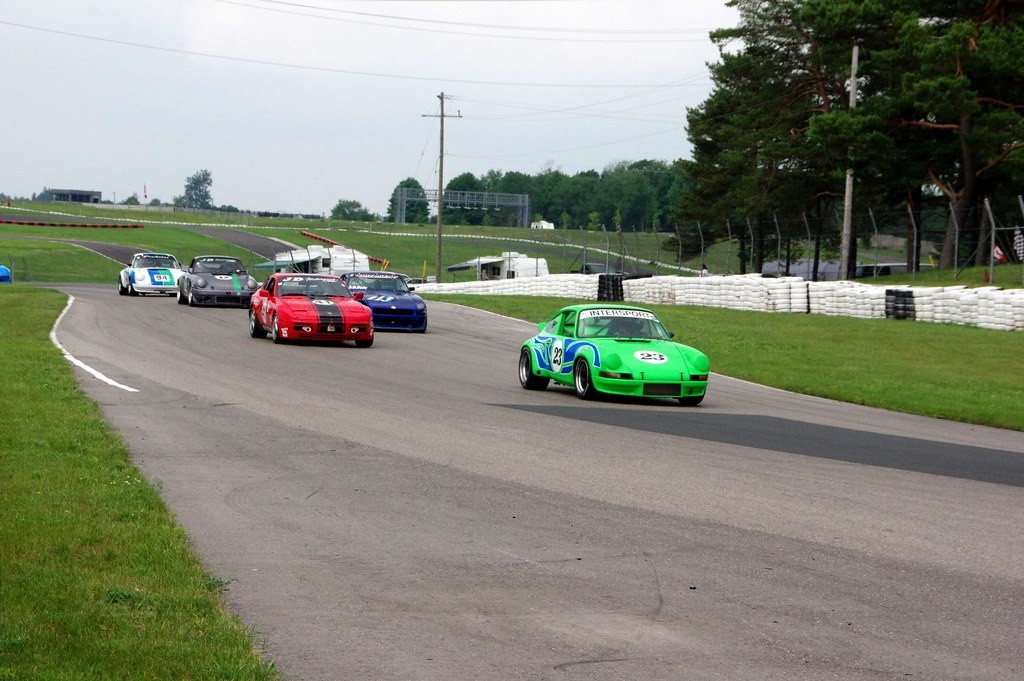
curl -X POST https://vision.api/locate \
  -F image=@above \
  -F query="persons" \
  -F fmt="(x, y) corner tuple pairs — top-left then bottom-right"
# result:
(699, 264), (708, 276)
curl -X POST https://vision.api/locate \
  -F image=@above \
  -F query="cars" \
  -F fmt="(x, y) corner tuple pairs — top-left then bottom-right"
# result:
(340, 270), (429, 332)
(173, 254), (258, 305)
(249, 272), (373, 347)
(0, 262), (10, 282)
(119, 252), (185, 296)
(515, 302), (709, 407)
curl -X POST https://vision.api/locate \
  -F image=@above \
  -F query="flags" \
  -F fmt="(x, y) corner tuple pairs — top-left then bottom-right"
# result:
(1014, 226), (1024, 261)
(993, 245), (1006, 263)
